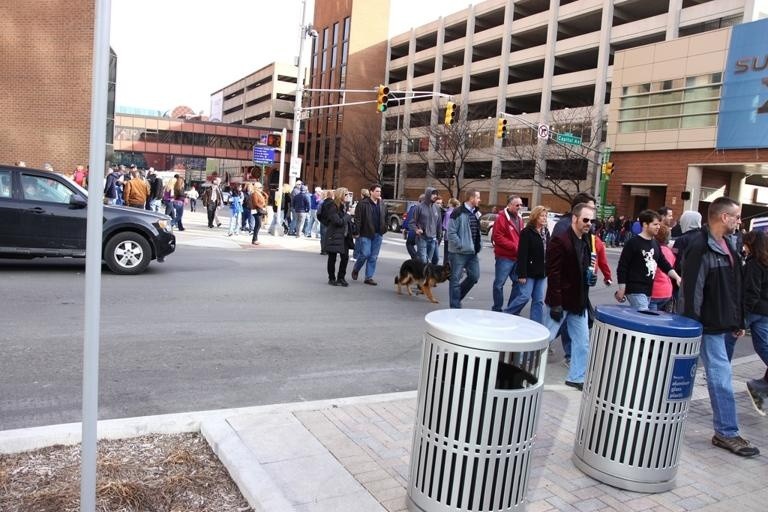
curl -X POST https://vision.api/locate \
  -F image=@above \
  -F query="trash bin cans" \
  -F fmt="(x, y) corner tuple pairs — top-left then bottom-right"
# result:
(405, 309), (551, 512)
(572, 304), (703, 494)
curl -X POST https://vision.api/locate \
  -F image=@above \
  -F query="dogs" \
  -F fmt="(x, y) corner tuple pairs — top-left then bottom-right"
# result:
(395, 259), (454, 303)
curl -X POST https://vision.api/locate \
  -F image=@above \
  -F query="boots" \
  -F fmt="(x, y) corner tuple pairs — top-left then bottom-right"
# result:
(179, 224), (184, 230)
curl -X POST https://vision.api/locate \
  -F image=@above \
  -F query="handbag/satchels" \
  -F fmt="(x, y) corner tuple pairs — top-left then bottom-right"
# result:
(258, 208), (267, 216)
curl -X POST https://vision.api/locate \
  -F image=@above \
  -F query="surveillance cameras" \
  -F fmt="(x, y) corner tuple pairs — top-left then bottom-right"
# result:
(311, 30), (318, 37)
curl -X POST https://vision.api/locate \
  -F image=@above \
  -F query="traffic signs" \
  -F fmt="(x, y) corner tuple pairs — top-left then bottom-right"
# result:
(253, 146), (274, 164)
(557, 134), (581, 146)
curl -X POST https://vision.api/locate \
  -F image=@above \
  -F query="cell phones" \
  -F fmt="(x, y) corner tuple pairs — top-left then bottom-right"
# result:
(615, 291), (626, 303)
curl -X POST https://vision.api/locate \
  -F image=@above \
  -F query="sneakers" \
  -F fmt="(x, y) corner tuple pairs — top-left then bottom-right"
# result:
(712, 432), (759, 455)
(745, 381), (766, 417)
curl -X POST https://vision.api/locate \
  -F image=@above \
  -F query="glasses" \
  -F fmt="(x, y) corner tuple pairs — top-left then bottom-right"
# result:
(583, 218), (596, 223)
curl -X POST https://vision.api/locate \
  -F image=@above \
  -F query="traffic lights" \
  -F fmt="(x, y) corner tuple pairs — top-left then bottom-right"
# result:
(376, 85), (393, 115)
(498, 119), (508, 141)
(606, 162), (617, 178)
(445, 101), (458, 127)
(265, 131), (282, 149)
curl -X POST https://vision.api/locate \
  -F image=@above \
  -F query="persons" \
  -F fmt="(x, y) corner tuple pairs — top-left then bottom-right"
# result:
(490, 192), (613, 391)
(606, 197), (768, 456)
(227, 171), (483, 309)
(4, 158), (223, 230)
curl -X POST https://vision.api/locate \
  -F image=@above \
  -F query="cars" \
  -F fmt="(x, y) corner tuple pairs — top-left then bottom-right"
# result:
(479, 213), (499, 235)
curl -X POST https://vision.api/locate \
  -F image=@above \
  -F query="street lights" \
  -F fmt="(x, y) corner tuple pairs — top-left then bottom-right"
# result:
(287, 26), (319, 210)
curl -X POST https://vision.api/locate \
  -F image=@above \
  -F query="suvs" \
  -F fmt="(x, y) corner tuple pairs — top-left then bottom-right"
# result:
(381, 198), (417, 233)
(0, 162), (177, 276)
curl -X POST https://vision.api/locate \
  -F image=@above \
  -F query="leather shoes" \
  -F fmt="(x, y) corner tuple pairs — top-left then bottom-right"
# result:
(328, 272), (377, 286)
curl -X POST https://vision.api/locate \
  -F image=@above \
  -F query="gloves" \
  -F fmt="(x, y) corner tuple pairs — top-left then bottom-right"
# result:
(589, 274), (597, 286)
(550, 305), (563, 323)
